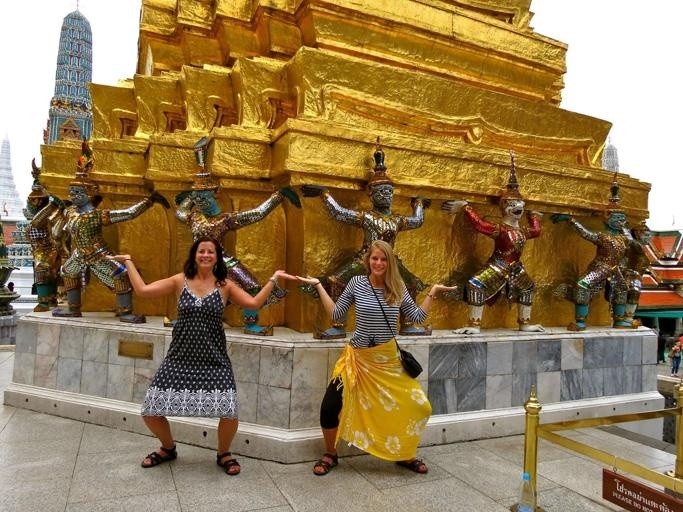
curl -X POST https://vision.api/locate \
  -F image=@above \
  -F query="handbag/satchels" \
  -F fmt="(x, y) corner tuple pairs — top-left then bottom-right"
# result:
(400, 350), (423, 378)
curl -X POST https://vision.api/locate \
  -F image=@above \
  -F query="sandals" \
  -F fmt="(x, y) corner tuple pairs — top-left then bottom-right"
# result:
(141, 444), (177, 468)
(217, 452), (240, 475)
(312, 453), (338, 475)
(397, 459), (428, 473)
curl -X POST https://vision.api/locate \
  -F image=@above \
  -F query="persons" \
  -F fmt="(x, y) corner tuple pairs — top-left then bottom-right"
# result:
(105, 238), (297, 476)
(297, 241), (458, 475)
(657, 331), (683, 376)
